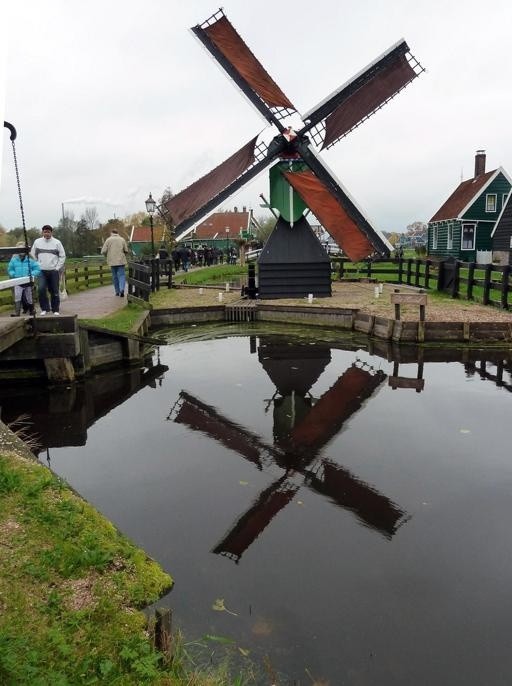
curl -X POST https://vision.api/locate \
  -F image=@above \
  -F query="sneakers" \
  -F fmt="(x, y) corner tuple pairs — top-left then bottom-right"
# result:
(40, 310), (60, 316)
(30, 313), (35, 316)
(10, 313), (21, 316)
(116, 293), (124, 297)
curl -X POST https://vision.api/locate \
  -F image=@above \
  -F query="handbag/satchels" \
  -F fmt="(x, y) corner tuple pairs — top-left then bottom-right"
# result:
(59, 289), (69, 301)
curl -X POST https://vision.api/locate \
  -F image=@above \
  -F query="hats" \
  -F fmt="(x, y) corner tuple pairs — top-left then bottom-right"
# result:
(16, 242), (31, 248)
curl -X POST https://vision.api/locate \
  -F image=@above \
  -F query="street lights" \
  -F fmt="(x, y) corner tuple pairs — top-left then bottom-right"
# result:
(225, 226), (230, 260)
(145, 191), (156, 294)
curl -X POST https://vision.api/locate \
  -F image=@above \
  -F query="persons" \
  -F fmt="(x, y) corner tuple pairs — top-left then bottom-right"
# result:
(7, 225), (66, 317)
(100, 229), (128, 298)
(158, 243), (238, 271)
